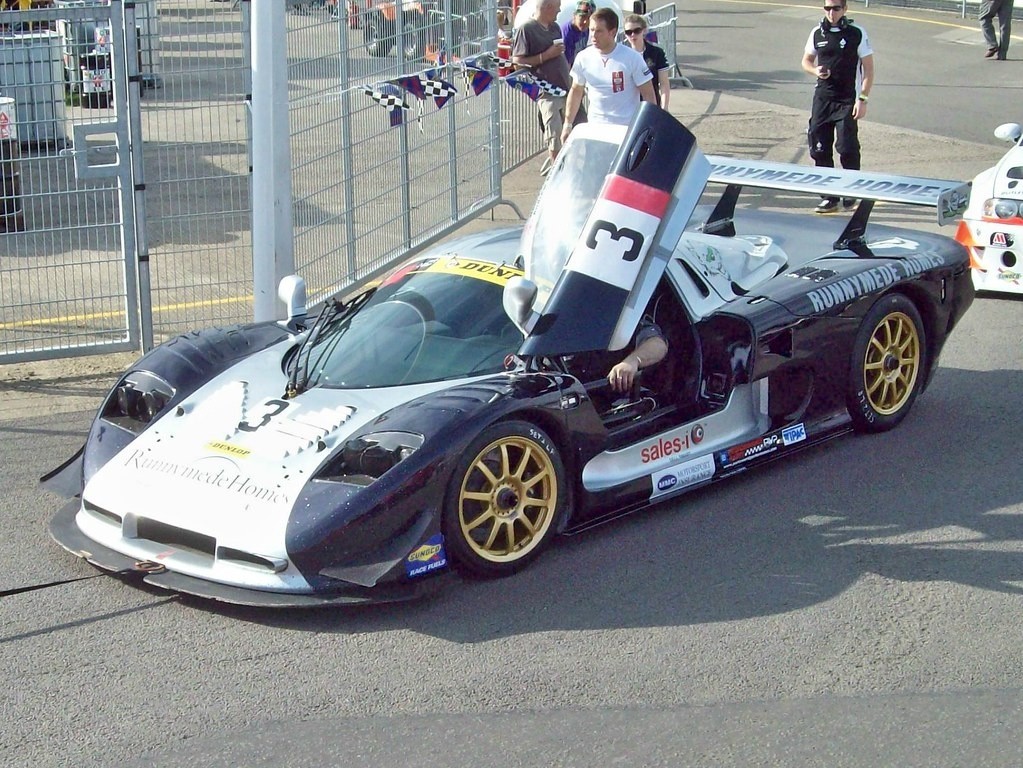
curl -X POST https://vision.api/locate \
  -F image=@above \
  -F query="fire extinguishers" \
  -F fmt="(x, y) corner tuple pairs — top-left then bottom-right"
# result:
(497, 34), (515, 77)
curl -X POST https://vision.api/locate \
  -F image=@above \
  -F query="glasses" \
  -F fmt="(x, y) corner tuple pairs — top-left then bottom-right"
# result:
(624, 27), (647, 36)
(824, 5), (846, 12)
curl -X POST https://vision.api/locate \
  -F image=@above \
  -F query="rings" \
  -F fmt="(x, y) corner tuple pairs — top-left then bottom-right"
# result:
(617, 377), (622, 380)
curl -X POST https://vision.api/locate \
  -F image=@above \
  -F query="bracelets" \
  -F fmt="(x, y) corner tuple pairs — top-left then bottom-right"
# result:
(563, 123), (572, 129)
(858, 93), (868, 103)
(539, 52), (543, 64)
(632, 352), (642, 369)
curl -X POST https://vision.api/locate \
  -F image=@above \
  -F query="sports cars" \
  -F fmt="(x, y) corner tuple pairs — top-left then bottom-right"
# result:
(959, 116), (1022, 299)
(38, 99), (980, 610)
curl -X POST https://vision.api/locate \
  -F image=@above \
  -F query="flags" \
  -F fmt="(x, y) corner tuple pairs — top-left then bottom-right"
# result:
(354, 52), (568, 135)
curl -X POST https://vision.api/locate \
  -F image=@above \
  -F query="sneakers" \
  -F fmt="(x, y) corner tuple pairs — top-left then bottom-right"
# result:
(843, 196), (857, 208)
(814, 199), (838, 213)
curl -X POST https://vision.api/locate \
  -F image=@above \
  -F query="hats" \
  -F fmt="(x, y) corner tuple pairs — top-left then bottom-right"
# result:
(572, 0), (597, 17)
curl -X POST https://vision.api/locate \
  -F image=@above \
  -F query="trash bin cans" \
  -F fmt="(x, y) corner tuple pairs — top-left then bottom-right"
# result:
(0, 96), (27, 233)
(81, 53), (112, 109)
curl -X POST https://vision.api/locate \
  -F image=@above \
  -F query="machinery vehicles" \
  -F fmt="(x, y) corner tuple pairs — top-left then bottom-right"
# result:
(342, 1), (517, 75)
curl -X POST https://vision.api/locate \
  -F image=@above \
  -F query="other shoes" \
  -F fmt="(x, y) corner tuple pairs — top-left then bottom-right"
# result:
(985, 47), (999, 58)
(993, 52), (1006, 60)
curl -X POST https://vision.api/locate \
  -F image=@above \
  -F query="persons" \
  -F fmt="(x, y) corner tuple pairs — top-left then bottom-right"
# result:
(802, 0), (874, 212)
(978, 0), (1014, 60)
(563, 319), (667, 415)
(511, 0), (670, 166)
